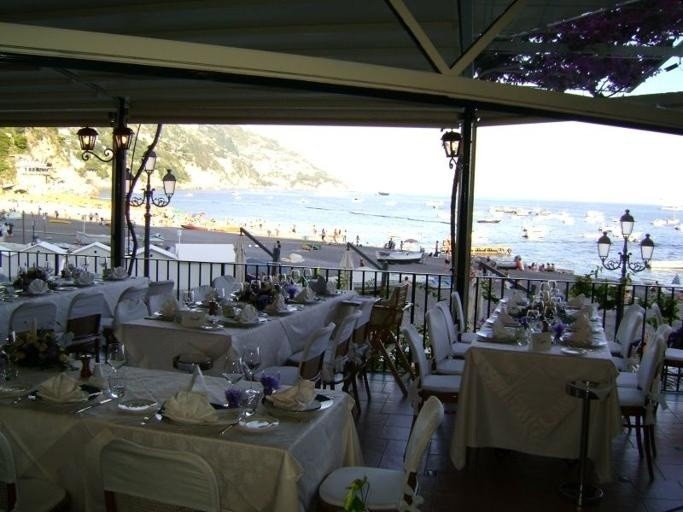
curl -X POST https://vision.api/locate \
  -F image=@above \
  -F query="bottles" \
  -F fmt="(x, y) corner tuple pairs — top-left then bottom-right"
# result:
(78, 354), (91, 379)
(93, 362), (104, 379)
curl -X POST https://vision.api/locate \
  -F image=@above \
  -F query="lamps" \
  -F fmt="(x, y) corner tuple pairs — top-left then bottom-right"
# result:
(77, 124), (114, 163)
(440, 124), (464, 168)
(111, 126), (135, 149)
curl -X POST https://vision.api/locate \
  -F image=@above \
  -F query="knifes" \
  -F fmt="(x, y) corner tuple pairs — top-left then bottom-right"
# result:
(140, 407), (159, 427)
(219, 410), (255, 437)
(72, 396), (112, 415)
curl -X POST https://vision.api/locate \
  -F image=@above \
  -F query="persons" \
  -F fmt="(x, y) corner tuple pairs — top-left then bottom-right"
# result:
(1, 194), (555, 272)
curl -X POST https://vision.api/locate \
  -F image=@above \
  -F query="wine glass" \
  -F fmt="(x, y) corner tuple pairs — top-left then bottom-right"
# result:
(104, 341), (128, 398)
(0, 328), (21, 378)
(259, 367), (279, 395)
(0, 257), (131, 303)
(155, 268), (344, 327)
(519, 279), (563, 351)
(219, 339), (261, 410)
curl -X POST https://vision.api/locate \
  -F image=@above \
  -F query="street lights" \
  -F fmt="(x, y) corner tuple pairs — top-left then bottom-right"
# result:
(31, 218), (36, 242)
(595, 207), (656, 340)
(42, 215), (47, 236)
(125, 144), (178, 278)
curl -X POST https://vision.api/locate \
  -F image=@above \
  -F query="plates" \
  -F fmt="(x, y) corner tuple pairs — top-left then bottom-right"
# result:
(236, 414), (280, 434)
(165, 403), (243, 428)
(559, 294), (608, 355)
(115, 396), (159, 413)
(0, 382), (31, 400)
(260, 391), (336, 418)
(475, 288), (530, 346)
(24, 382), (103, 406)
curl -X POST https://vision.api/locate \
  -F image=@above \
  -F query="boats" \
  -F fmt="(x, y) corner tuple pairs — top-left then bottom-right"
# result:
(493, 255), (523, 270)
(178, 223), (208, 232)
(476, 207), (550, 224)
(378, 192), (390, 195)
(75, 230), (166, 245)
(645, 259), (683, 269)
(49, 218), (72, 224)
(441, 237), (508, 259)
(376, 248), (424, 263)
